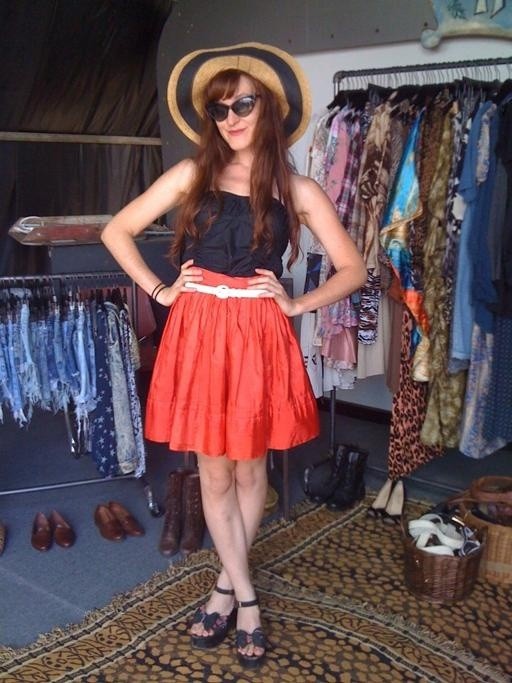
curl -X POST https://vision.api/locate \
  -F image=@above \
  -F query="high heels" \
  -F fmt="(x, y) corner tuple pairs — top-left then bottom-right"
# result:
(382, 478), (407, 526)
(368, 477), (396, 519)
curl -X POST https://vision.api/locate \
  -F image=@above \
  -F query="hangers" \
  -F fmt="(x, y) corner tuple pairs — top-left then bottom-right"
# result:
(330, 51), (511, 100)
(1, 261), (136, 317)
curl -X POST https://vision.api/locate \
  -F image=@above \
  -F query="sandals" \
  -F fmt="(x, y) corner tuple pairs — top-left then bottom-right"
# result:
(236, 598), (267, 670)
(188, 586), (236, 646)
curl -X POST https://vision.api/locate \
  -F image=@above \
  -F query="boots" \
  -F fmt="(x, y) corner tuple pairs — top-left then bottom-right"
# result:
(160, 471), (188, 557)
(178, 473), (206, 554)
(311, 443), (360, 503)
(325, 449), (368, 513)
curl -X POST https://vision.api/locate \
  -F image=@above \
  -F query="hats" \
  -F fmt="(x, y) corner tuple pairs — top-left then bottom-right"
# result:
(166, 42), (311, 148)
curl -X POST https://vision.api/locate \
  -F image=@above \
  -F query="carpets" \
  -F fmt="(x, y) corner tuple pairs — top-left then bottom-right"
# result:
(0, 484), (511, 681)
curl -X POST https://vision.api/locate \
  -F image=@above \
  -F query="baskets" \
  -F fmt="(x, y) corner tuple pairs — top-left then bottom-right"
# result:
(401, 521), (488, 604)
(451, 476), (512, 588)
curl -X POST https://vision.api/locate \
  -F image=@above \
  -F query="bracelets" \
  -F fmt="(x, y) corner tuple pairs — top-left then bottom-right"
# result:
(154, 286), (167, 301)
(150, 281), (165, 298)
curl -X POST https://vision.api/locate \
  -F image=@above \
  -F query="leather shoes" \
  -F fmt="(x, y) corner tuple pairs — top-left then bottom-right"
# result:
(49, 510), (74, 548)
(108, 501), (144, 536)
(93, 505), (125, 542)
(31, 513), (52, 551)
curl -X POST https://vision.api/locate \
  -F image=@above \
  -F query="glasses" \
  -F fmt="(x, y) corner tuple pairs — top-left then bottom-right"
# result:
(206, 95), (259, 121)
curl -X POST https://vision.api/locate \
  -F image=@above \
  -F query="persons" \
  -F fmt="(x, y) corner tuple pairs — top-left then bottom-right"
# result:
(99, 67), (369, 668)
